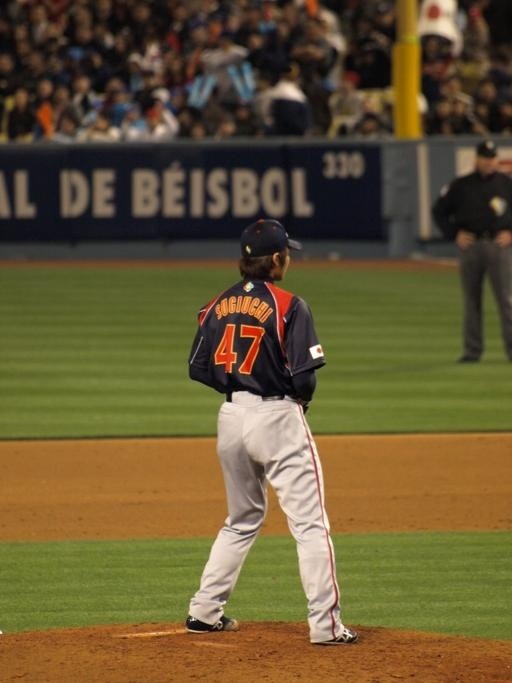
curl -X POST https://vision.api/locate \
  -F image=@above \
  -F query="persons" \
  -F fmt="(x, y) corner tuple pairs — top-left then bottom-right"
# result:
(429, 138), (511, 365)
(183, 215), (360, 646)
(1, 0), (512, 146)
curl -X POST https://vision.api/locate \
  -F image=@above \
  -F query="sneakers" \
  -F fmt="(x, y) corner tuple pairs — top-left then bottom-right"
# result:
(308, 626), (361, 645)
(184, 610), (242, 633)
(456, 355), (480, 363)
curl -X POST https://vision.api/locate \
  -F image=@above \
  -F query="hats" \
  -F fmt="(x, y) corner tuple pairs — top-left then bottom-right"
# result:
(240, 217), (302, 255)
(476, 138), (498, 160)
